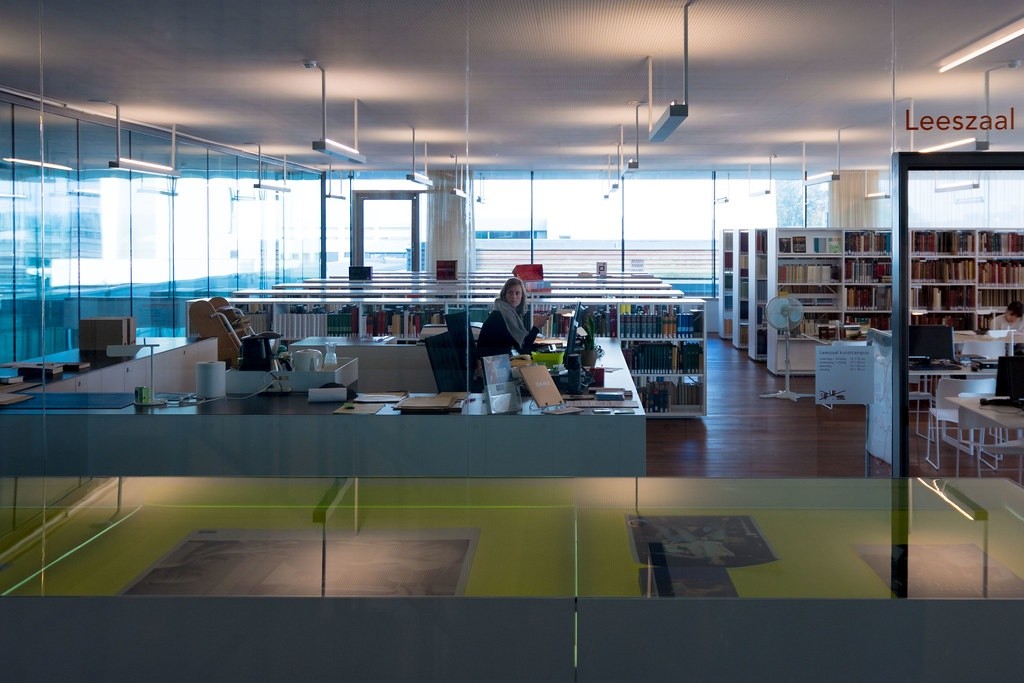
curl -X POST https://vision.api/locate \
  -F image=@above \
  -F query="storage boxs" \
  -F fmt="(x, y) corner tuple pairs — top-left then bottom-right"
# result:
(78, 316), (136, 350)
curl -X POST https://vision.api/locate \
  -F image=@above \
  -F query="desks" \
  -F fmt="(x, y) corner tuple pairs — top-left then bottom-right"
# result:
(942, 397), (1024, 430)
(878, 335), (1024, 349)
(904, 358), (1022, 376)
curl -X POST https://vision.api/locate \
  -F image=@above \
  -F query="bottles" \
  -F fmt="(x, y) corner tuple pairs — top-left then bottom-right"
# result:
(323, 342), (337, 371)
(568, 353), (581, 395)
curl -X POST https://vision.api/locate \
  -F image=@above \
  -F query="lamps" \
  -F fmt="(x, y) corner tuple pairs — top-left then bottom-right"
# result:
(95, 0), (1024, 202)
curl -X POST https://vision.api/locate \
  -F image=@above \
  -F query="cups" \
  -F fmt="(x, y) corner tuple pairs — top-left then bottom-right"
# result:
(590, 366), (605, 386)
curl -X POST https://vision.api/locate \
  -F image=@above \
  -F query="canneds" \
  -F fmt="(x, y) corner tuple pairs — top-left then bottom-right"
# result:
(134, 386), (149, 404)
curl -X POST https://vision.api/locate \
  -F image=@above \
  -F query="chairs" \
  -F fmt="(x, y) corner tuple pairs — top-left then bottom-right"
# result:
(424, 332), (479, 394)
(907, 342), (1024, 485)
(446, 313), (477, 356)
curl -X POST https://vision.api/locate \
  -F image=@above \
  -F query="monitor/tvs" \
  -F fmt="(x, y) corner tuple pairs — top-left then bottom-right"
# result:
(563, 302), (595, 394)
(996, 356), (1024, 400)
(910, 325), (956, 365)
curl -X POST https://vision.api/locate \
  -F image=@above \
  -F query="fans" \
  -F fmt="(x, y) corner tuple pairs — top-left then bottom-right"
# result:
(758, 296), (817, 401)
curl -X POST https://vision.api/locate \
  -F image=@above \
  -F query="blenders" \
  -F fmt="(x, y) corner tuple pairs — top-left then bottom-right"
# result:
(258, 330), (293, 371)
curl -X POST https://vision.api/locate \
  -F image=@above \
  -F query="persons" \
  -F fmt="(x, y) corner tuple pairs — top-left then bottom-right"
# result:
(990, 299), (1024, 331)
(479, 277), (549, 379)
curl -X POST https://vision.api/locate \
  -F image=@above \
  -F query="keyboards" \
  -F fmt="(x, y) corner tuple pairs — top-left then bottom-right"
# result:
(988, 398), (1024, 407)
(909, 364), (961, 370)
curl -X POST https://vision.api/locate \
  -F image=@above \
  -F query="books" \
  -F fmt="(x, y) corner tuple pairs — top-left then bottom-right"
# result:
(636, 381), (704, 414)
(533, 305), (703, 339)
(0, 362), (88, 385)
(977, 230), (1024, 335)
(773, 237), (843, 342)
(78, 315), (135, 361)
(242, 312), (272, 334)
(277, 306), (443, 339)
(740, 232), (749, 345)
(843, 231), (894, 333)
(724, 233), (733, 337)
(911, 230), (975, 332)
(624, 341), (701, 374)
(521, 363), (564, 408)
(757, 231), (767, 356)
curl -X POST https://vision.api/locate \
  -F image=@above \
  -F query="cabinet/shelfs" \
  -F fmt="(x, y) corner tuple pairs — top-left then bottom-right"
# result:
(187, 274), (709, 418)
(718, 230), (1024, 376)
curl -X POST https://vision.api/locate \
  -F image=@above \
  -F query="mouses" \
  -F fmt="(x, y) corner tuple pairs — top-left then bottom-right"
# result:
(973, 366), (979, 371)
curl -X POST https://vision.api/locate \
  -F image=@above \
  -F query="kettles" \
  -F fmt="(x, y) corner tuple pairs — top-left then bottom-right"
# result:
(293, 348), (324, 372)
